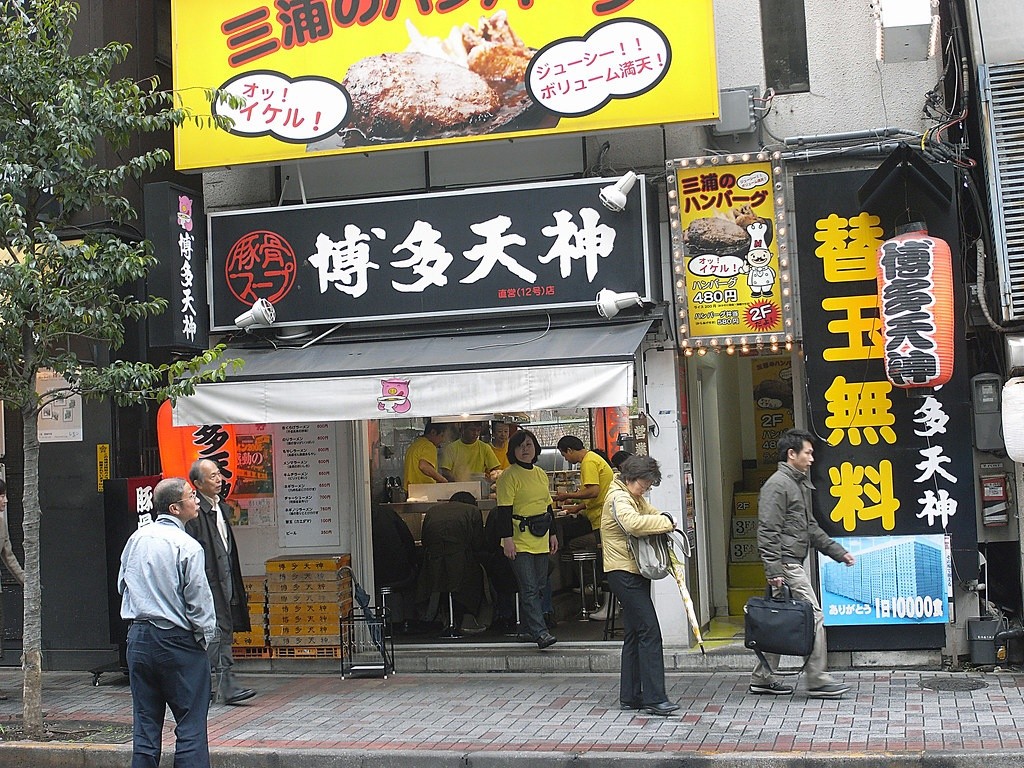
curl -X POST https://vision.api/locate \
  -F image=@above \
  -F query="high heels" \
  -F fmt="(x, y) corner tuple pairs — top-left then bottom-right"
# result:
(643, 702), (678, 714)
(619, 700), (645, 710)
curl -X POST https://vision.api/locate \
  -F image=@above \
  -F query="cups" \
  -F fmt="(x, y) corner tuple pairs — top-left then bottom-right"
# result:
(557, 487), (567, 494)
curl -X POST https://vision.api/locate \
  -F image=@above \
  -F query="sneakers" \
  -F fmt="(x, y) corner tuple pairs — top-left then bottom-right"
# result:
(749, 680), (793, 695)
(806, 680), (850, 696)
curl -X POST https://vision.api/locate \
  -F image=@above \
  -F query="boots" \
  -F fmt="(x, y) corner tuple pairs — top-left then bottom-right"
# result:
(589, 590), (619, 620)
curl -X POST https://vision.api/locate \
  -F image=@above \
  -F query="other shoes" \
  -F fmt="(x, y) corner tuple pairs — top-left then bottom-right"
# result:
(461, 614), (486, 633)
(544, 614), (557, 628)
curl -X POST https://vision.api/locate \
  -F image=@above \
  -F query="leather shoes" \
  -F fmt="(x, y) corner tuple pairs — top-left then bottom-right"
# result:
(225, 687), (255, 705)
(538, 633), (556, 649)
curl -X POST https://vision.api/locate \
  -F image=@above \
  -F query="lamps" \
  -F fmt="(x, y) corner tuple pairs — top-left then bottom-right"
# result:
(233, 297), (276, 335)
(596, 288), (645, 321)
(598, 170), (638, 213)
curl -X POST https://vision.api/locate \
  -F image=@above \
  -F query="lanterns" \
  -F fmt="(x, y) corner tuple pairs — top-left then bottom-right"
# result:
(878, 222), (954, 398)
(157, 398), (238, 502)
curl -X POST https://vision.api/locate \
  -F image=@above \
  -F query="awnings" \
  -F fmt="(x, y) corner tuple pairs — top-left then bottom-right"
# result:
(172, 322), (652, 427)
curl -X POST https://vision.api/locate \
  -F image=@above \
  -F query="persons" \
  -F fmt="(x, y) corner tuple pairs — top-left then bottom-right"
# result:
(748, 429), (855, 697)
(184, 459), (257, 703)
(496, 429), (559, 649)
(601, 456), (679, 714)
(403, 418), (512, 497)
(551, 435), (633, 593)
(116, 478), (217, 768)
(419, 492), (488, 633)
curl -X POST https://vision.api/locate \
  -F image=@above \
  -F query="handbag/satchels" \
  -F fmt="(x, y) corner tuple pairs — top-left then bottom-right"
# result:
(743, 582), (814, 656)
(513, 512), (552, 537)
(614, 495), (691, 580)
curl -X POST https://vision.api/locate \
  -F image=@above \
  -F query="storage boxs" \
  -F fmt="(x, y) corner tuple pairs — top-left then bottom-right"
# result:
(232, 554), (351, 648)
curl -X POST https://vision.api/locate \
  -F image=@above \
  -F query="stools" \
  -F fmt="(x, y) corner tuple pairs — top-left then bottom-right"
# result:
(380, 550), (626, 640)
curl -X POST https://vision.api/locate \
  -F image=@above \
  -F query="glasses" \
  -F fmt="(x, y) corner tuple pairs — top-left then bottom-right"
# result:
(173, 491), (198, 504)
(634, 478), (652, 493)
